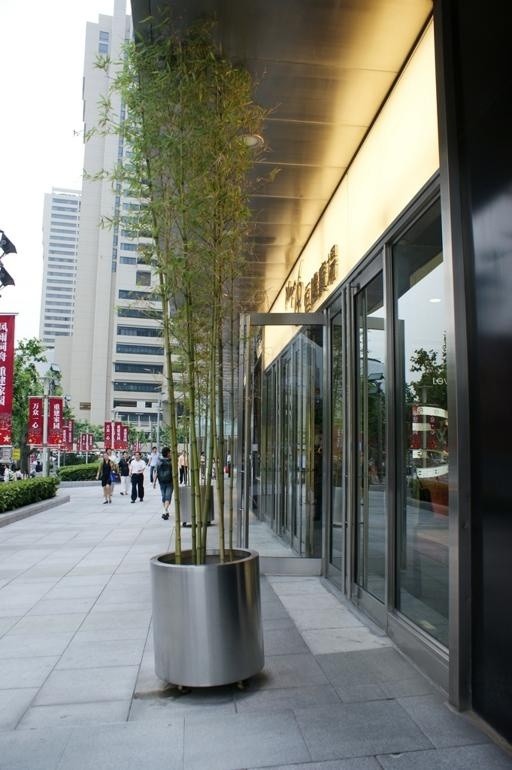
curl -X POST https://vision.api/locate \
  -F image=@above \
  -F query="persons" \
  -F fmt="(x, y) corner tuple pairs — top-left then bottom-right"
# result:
(96, 448), (146, 504)
(147, 447), (234, 520)
(4, 464), (37, 482)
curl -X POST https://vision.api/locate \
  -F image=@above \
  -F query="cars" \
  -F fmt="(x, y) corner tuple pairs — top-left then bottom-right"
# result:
(0, 461), (16, 481)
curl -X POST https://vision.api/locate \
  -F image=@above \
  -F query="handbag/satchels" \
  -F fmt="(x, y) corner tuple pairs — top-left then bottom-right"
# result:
(110, 472), (121, 483)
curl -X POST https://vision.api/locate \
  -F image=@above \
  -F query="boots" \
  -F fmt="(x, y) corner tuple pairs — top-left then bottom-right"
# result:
(140, 498), (143, 502)
(120, 492), (123, 495)
(103, 500), (111, 504)
(130, 499), (135, 503)
(162, 513), (169, 520)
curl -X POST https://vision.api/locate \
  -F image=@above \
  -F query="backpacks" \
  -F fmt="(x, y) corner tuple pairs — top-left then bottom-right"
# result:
(157, 458), (172, 486)
